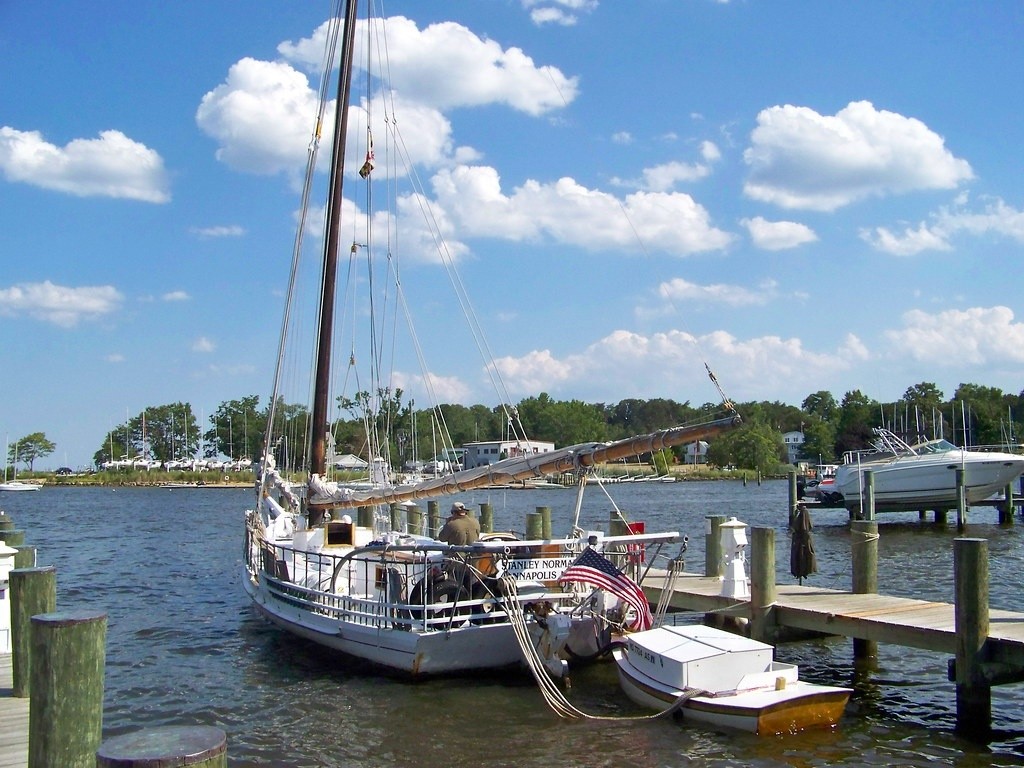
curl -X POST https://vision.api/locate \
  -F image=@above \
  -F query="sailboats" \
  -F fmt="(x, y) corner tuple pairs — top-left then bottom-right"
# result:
(100, 406), (252, 470)
(0, 432), (43, 491)
(241, 1), (745, 680)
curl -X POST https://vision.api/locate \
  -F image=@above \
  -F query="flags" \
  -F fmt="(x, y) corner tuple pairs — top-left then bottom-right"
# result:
(557, 546), (653, 632)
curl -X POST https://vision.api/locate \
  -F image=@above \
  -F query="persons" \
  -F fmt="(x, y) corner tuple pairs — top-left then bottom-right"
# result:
(439, 502), (480, 629)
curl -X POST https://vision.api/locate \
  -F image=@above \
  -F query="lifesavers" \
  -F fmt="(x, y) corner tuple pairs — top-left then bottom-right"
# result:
(470, 574), (520, 625)
(409, 569), (471, 630)
(225, 476), (229, 480)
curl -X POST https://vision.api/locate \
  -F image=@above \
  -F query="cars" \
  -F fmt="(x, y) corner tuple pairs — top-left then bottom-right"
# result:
(55, 467), (72, 475)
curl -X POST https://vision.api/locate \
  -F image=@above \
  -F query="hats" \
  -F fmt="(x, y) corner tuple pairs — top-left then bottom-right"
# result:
(451, 502), (465, 512)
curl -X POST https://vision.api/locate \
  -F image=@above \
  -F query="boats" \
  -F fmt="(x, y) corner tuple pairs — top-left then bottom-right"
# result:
(797, 400), (1024, 502)
(611, 625), (853, 735)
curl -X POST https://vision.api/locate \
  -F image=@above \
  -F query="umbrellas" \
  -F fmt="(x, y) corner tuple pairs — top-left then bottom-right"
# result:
(791, 506), (817, 586)
